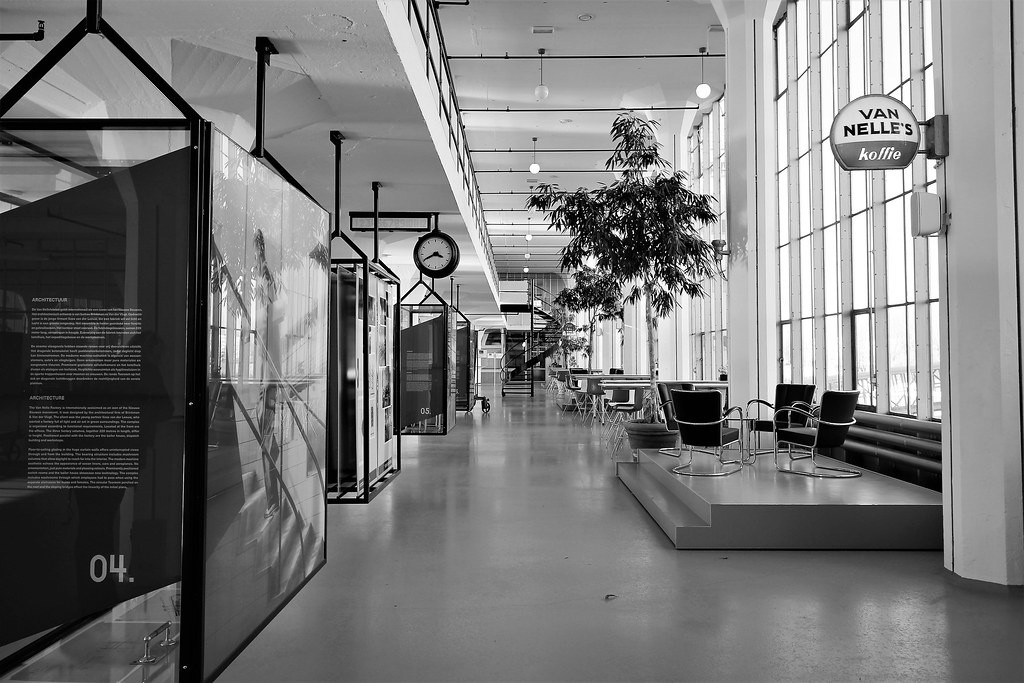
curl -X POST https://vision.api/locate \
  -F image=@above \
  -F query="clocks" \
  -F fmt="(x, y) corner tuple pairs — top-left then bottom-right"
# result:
(413, 212), (461, 279)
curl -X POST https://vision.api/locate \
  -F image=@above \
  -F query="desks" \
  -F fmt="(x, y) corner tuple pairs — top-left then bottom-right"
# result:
(571, 374), (650, 380)
(600, 379), (728, 384)
(599, 384), (728, 389)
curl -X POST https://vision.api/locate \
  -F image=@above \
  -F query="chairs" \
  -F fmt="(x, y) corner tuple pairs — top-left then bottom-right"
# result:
(546, 366), (863, 479)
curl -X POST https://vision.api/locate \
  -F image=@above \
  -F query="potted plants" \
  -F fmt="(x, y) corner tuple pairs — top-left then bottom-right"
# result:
(522, 107), (722, 463)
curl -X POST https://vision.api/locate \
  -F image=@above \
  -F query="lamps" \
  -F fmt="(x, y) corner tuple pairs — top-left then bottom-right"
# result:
(534, 49), (549, 102)
(696, 47), (711, 98)
(523, 137), (540, 273)
